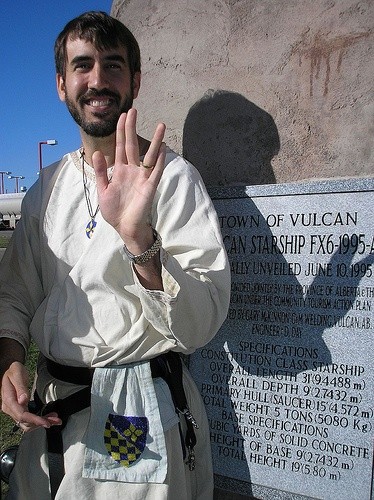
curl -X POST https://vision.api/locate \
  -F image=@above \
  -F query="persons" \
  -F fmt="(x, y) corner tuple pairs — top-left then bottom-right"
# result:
(0, 11), (232, 500)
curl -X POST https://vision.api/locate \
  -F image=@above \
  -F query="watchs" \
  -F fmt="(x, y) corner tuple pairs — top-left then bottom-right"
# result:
(123, 227), (163, 264)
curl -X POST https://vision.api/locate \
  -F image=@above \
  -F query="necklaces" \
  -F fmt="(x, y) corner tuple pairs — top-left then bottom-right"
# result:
(80, 144), (113, 238)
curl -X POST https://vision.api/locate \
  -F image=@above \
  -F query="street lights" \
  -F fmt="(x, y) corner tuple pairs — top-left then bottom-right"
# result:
(0, 171), (12, 194)
(37, 140), (58, 175)
(8, 175), (24, 193)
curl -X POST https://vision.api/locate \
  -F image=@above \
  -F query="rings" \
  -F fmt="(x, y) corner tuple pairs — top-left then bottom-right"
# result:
(142, 159), (154, 169)
(16, 420), (23, 427)
